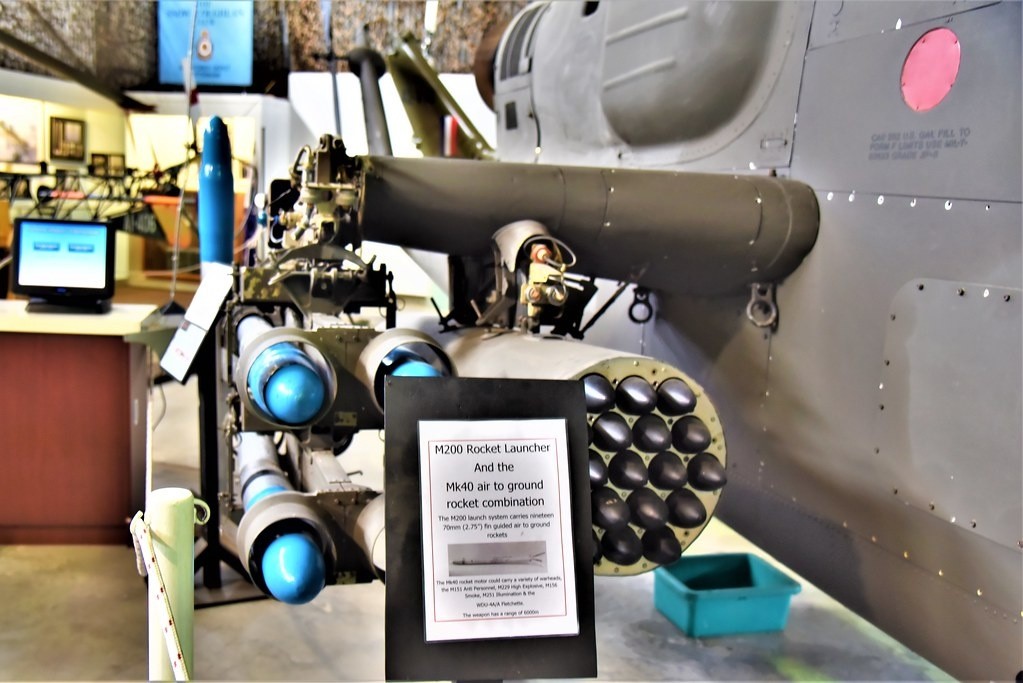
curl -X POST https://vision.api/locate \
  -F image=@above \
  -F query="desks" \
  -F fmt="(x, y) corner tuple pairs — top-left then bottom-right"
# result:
(0, 298), (158, 544)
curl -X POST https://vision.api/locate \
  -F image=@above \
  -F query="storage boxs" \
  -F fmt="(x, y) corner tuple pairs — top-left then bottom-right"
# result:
(653, 552), (802, 637)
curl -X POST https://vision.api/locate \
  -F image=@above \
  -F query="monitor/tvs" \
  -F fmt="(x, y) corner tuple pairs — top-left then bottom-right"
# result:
(49, 117), (88, 166)
(10, 218), (115, 315)
